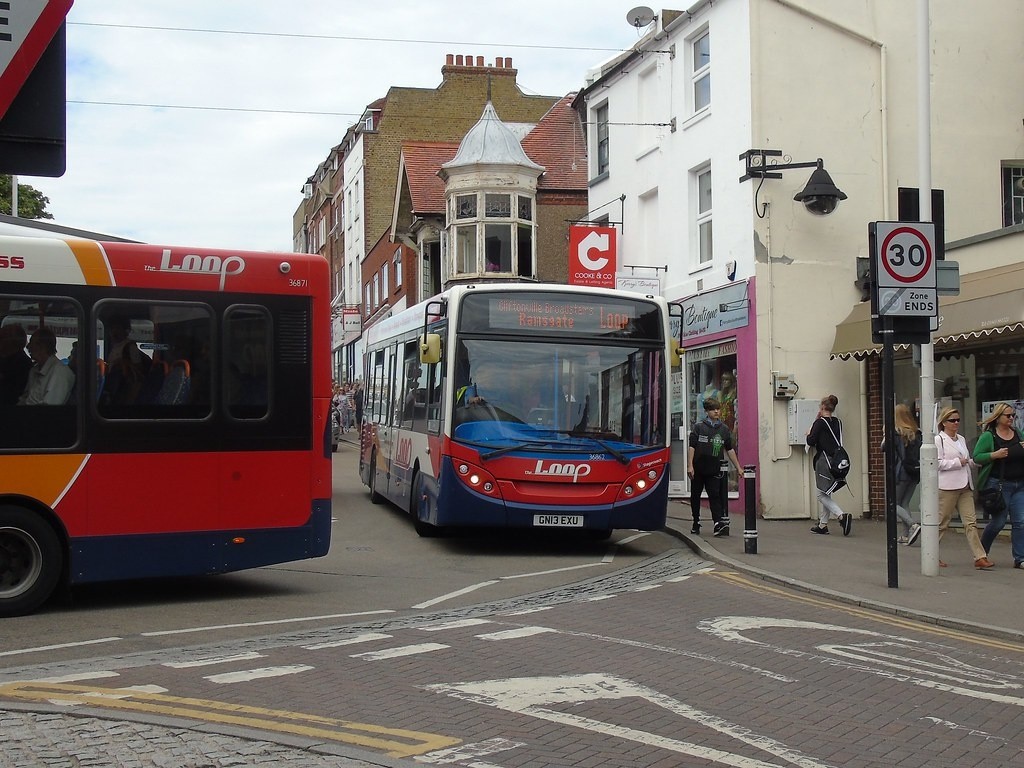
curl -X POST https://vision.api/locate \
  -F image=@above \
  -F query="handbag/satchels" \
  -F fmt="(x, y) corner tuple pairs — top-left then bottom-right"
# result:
(981, 492), (1006, 514)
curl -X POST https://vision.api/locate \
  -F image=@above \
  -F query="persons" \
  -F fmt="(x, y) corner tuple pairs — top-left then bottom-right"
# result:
(934, 407), (995, 570)
(972, 403), (1024, 569)
(0, 313), (153, 416)
(688, 398), (743, 536)
(881, 404), (921, 546)
(804, 395), (852, 536)
(697, 369), (738, 481)
(465, 362), (514, 411)
(332, 375), (363, 440)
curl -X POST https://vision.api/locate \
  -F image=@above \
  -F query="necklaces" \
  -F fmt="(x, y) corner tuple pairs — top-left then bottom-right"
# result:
(995, 426), (1014, 440)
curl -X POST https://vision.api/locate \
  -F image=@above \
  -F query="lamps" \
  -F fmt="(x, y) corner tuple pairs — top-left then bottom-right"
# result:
(331, 303), (380, 324)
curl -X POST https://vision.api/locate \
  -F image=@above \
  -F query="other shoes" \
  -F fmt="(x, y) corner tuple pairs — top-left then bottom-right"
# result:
(975, 558), (995, 570)
(1013, 561), (1024, 569)
(939, 560), (947, 567)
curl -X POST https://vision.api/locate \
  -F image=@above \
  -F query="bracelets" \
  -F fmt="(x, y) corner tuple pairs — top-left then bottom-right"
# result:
(883, 434), (885, 437)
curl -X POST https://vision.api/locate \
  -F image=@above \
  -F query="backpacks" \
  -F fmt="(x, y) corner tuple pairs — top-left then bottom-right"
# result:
(896, 429), (923, 485)
(819, 417), (850, 479)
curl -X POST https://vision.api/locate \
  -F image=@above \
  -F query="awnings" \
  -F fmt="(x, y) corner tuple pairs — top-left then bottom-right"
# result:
(829, 262), (1024, 362)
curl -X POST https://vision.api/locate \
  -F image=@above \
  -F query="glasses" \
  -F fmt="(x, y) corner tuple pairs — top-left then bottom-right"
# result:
(1001, 413), (1015, 418)
(945, 418), (960, 423)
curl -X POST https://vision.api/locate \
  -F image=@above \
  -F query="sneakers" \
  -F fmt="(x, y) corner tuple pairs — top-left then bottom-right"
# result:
(908, 523), (921, 544)
(713, 521), (728, 536)
(810, 518), (829, 534)
(839, 513), (852, 535)
(691, 521), (702, 533)
(897, 536), (909, 546)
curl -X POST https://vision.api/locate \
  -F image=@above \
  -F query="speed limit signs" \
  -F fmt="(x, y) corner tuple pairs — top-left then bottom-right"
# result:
(881, 227), (931, 284)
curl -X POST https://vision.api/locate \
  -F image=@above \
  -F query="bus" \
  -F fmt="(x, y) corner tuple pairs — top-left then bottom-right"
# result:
(0, 214), (333, 618)
(359, 278), (685, 538)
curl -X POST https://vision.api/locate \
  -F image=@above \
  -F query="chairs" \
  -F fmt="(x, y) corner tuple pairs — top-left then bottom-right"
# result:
(154, 359), (190, 405)
(138, 361), (168, 405)
(96, 358), (105, 403)
